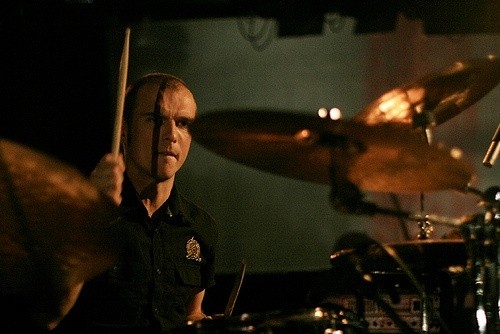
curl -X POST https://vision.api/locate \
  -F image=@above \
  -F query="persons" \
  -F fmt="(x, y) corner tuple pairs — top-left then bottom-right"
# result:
(44, 73), (224, 334)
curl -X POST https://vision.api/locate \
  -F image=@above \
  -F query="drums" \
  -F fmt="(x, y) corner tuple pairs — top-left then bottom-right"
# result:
(175, 305), (373, 334)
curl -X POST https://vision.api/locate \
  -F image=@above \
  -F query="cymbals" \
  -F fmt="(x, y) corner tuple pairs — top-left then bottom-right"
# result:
(0, 134), (130, 301)
(183, 107), (478, 195)
(327, 237), (467, 278)
(348, 53), (500, 132)
